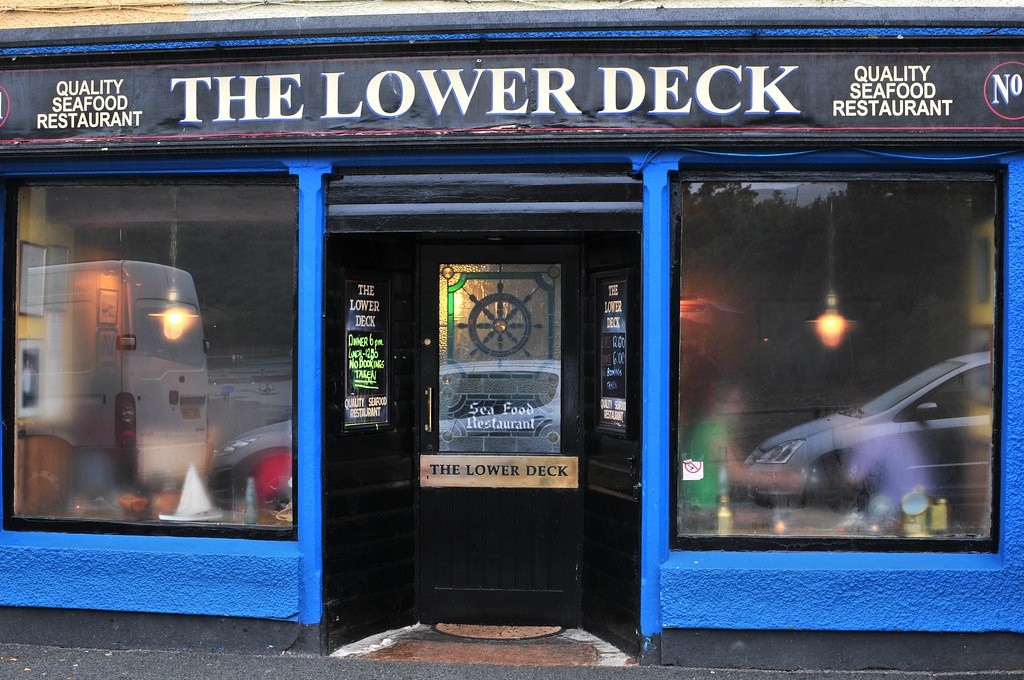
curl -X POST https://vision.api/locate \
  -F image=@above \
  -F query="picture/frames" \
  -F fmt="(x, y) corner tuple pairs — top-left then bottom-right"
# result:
(18, 338), (44, 418)
(96, 288), (119, 406)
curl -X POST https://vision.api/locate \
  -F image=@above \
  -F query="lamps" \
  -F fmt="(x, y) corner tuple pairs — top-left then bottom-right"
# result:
(802, 180), (858, 347)
(147, 187), (200, 343)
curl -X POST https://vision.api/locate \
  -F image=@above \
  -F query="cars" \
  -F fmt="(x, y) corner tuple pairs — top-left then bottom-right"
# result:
(741, 351), (993, 525)
(212, 413), (293, 527)
(440, 359), (566, 455)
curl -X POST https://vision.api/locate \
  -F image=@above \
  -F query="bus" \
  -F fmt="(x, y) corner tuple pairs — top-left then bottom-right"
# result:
(16, 260), (212, 516)
